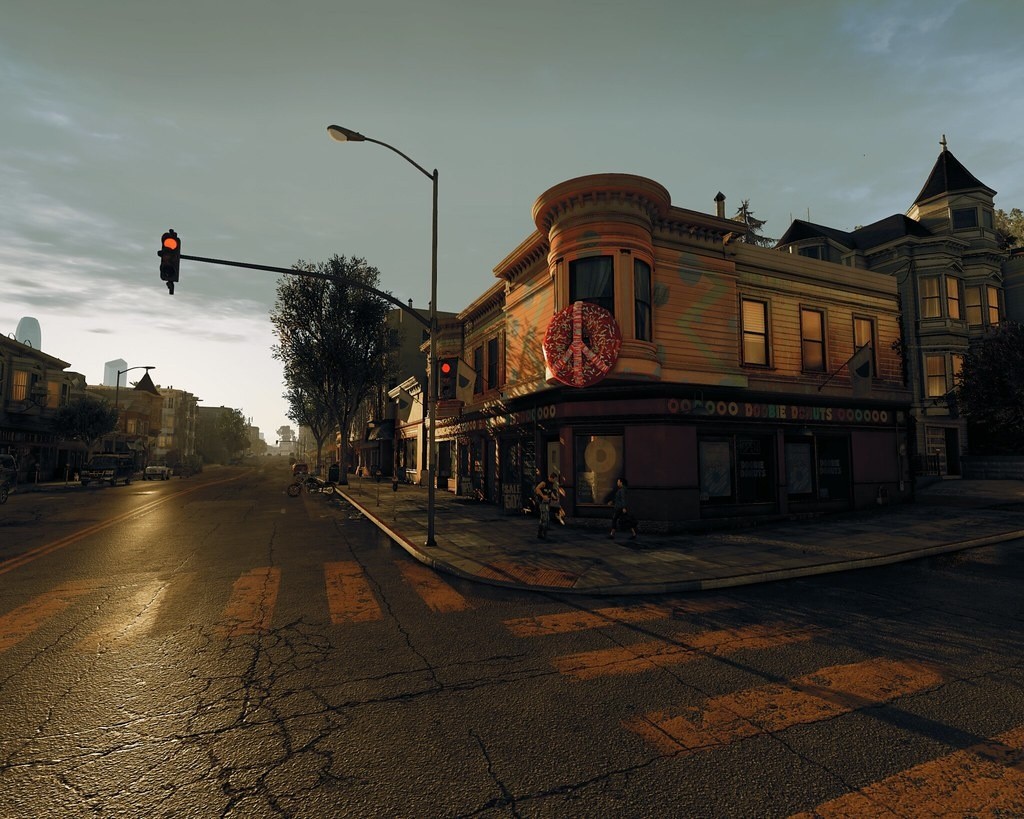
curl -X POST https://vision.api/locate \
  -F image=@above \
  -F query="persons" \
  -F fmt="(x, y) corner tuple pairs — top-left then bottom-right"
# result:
(531, 467), (548, 519)
(535, 472), (559, 539)
(605, 477), (637, 540)
(473, 465), (486, 502)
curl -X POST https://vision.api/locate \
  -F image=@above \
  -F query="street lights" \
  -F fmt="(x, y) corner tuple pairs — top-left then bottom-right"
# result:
(326, 124), (439, 547)
(276, 440), (305, 461)
(111, 366), (161, 453)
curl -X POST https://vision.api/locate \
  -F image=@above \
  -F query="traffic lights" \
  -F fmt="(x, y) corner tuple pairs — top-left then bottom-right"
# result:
(159, 229), (181, 281)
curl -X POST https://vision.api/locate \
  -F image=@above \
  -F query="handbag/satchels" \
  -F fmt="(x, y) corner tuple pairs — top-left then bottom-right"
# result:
(618, 511), (638, 531)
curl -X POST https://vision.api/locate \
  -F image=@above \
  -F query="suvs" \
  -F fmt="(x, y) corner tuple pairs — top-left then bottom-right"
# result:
(79, 454), (133, 487)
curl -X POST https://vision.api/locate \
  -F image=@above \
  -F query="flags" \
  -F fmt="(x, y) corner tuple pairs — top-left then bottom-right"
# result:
(946, 381), (972, 420)
(455, 358), (477, 404)
(397, 389), (414, 423)
(846, 342), (874, 405)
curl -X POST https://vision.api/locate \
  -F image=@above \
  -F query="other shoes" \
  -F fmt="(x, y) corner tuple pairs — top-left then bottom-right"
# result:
(606, 535), (616, 540)
(628, 534), (637, 540)
(543, 532), (549, 540)
(536, 528), (543, 538)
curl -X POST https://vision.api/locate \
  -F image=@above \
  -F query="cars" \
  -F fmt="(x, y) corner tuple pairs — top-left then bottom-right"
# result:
(293, 463), (307, 477)
(146, 460), (172, 481)
(0, 454), (17, 504)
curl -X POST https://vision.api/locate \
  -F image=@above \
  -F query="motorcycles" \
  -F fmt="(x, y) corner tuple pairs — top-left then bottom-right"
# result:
(287, 473), (334, 497)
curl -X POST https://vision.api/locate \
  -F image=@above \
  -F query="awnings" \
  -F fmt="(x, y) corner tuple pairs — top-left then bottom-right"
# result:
(368, 422), (395, 440)
